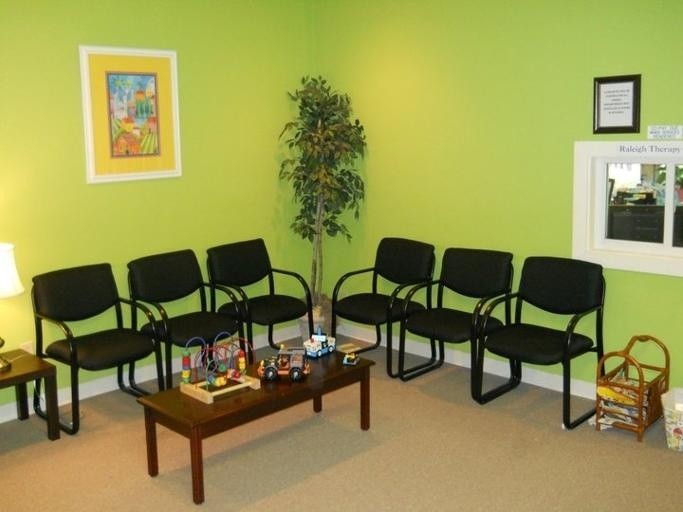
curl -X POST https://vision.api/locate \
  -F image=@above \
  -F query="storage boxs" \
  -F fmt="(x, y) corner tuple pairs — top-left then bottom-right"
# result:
(661, 388), (683, 451)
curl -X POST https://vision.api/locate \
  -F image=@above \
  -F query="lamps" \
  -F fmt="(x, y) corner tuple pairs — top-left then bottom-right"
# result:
(0, 242), (27, 373)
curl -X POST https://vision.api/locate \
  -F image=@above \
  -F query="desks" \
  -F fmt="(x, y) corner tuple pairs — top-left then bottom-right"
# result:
(0, 348), (62, 441)
(137, 350), (376, 505)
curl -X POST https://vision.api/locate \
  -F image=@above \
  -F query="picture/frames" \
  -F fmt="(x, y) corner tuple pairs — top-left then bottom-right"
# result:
(592, 73), (640, 135)
(79, 46), (181, 185)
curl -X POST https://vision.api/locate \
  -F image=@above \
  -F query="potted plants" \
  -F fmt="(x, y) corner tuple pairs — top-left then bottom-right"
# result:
(279, 76), (367, 344)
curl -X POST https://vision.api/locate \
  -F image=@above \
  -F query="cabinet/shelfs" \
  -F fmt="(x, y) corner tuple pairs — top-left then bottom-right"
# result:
(596, 335), (670, 441)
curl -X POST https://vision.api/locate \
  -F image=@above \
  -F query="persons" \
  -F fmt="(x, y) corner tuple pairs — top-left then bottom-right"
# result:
(637, 176), (647, 187)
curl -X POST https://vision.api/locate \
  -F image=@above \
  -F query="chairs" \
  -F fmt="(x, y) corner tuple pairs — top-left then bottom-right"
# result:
(398, 248), (517, 399)
(127, 249), (245, 396)
(331, 238), (436, 378)
(31, 263), (165, 434)
(476, 257), (607, 429)
(206, 238), (314, 364)
